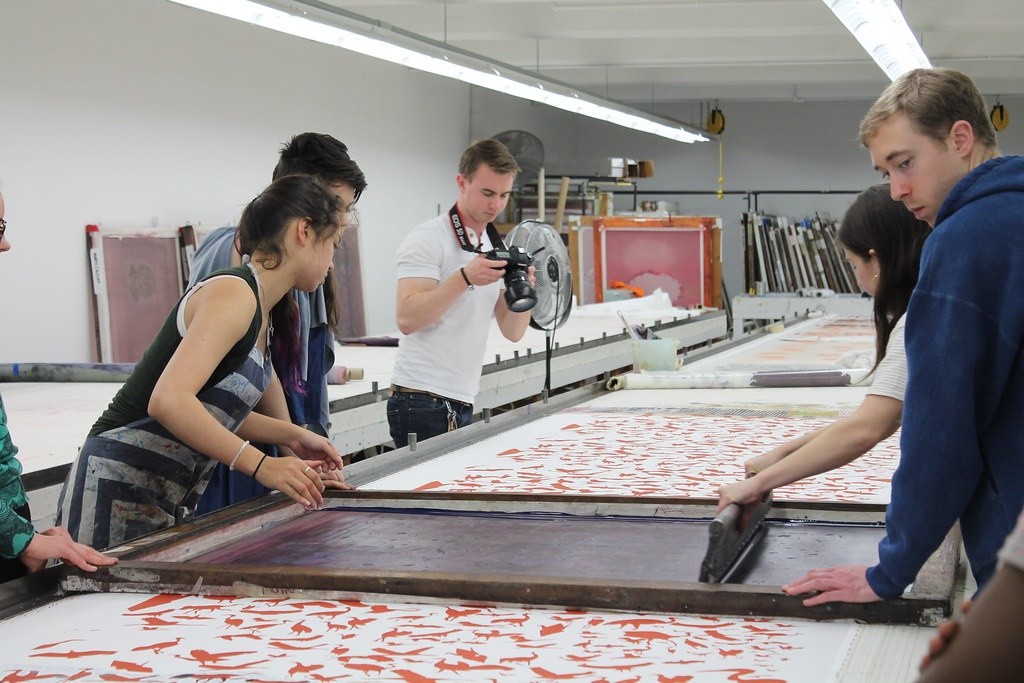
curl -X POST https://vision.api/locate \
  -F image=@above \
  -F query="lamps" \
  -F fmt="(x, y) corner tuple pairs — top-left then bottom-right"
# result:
(167, 0), (719, 145)
(822, 0), (932, 82)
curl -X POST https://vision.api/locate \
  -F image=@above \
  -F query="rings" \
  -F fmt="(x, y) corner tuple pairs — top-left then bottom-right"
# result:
(304, 467), (311, 475)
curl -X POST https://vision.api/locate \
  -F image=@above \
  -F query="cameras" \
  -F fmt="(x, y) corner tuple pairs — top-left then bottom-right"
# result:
(485, 244), (537, 313)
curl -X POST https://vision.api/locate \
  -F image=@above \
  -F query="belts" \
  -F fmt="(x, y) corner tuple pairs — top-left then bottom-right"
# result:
(390, 385), (470, 407)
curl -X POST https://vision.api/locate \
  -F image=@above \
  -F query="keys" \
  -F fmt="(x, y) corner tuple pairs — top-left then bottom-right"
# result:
(444, 401), (458, 432)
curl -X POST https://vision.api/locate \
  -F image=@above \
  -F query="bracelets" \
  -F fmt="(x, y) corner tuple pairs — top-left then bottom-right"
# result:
(229, 441), (250, 470)
(460, 267), (475, 292)
(252, 454), (269, 479)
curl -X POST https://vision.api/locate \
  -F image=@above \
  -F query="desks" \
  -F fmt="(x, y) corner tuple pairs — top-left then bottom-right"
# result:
(0, 308), (963, 683)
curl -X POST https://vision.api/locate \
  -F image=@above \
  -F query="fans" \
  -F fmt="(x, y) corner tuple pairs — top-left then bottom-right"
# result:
(506, 220), (574, 399)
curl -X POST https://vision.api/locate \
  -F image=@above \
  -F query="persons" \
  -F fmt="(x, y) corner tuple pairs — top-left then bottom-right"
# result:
(54, 173), (344, 563)
(782, 71), (1024, 606)
(714, 182), (933, 515)
(390, 139), (535, 448)
(197, 131), (356, 512)
(913, 510), (1024, 683)
(0, 193), (119, 573)
